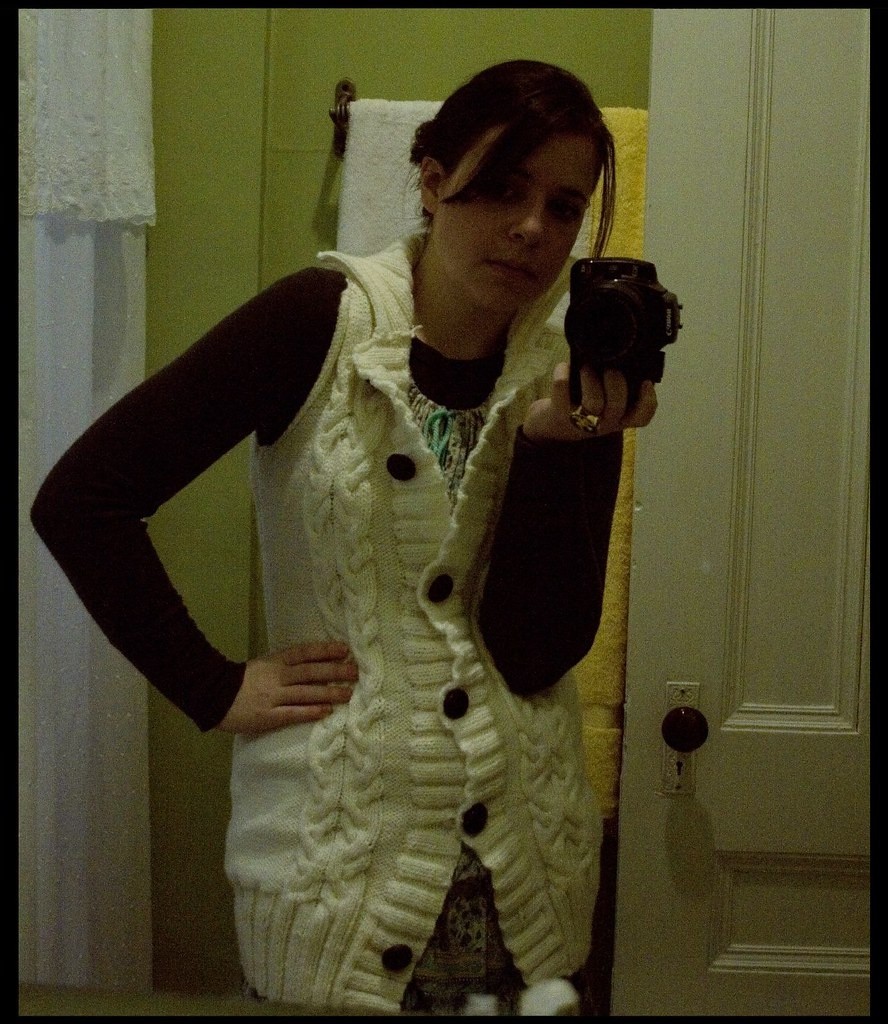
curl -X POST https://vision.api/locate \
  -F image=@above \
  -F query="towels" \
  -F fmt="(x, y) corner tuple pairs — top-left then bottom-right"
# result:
(334, 101), (649, 820)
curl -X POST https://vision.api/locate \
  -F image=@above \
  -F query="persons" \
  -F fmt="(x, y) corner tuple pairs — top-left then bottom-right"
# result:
(30, 56), (658, 1014)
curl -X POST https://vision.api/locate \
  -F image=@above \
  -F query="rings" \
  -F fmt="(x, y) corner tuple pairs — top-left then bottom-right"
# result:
(570, 405), (601, 435)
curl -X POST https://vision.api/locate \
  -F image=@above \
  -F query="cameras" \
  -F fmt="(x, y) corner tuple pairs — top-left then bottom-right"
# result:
(565, 256), (683, 402)
